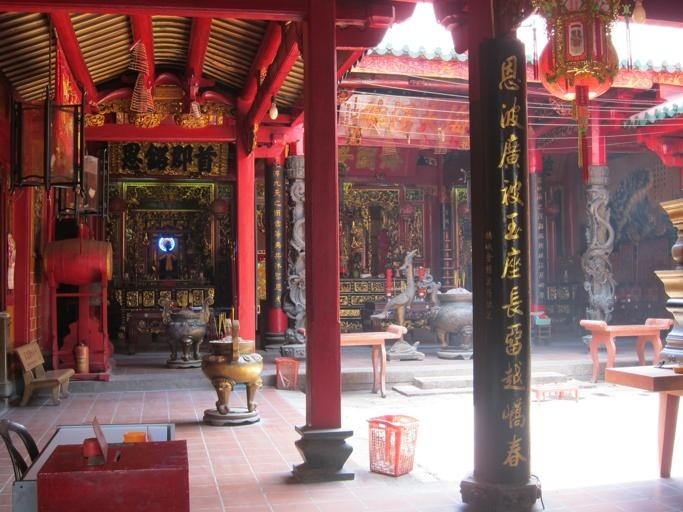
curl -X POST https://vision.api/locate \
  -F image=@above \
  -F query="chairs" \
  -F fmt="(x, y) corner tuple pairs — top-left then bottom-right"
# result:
(0, 419), (40, 482)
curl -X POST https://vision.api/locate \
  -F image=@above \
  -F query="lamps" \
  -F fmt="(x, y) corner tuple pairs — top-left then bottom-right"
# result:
(269, 95), (279, 120)
(631, 0), (646, 25)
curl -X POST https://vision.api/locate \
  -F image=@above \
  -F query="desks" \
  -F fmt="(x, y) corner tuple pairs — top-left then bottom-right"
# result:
(126, 310), (217, 355)
(604, 366), (683, 478)
(11, 423), (176, 512)
(296, 325), (408, 398)
(580, 318), (675, 384)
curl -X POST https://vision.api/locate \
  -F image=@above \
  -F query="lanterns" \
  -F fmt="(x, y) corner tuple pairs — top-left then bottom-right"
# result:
(537, 36), (618, 168)
(529, 1), (627, 184)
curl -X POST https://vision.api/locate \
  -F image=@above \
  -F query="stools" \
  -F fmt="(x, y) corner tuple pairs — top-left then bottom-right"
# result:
(536, 325), (553, 345)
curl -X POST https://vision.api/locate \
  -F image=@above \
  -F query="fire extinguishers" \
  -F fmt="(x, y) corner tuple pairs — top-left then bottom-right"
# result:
(74, 340), (89, 373)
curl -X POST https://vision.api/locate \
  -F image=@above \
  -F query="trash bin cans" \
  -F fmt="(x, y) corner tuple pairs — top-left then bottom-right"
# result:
(366, 414), (418, 476)
(273, 357), (299, 390)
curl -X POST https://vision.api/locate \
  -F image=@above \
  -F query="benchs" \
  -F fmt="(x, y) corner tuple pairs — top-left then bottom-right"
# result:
(13, 342), (75, 406)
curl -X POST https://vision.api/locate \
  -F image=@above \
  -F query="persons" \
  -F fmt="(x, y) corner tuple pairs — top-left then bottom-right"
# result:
(357, 299), (408, 343)
